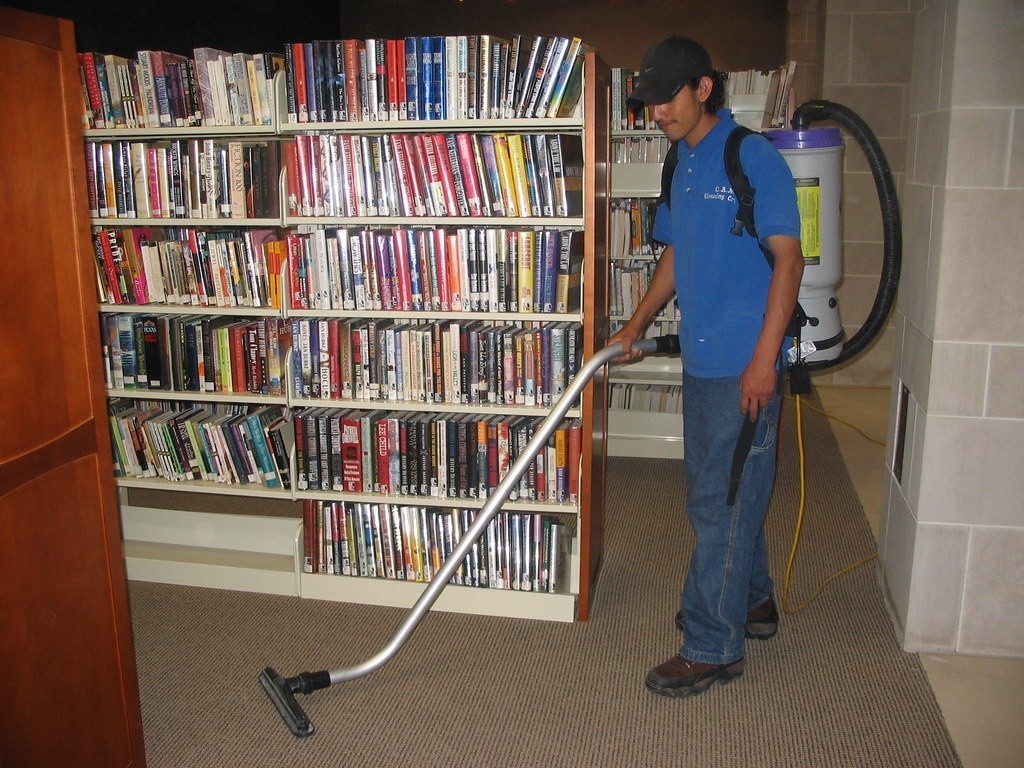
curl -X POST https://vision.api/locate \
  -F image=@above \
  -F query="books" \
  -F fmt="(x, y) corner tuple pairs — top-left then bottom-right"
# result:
(79, 34), (797, 594)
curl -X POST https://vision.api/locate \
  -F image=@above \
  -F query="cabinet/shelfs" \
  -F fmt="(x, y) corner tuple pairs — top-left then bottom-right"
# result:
(83, 52), (612, 625)
(605, 89), (796, 459)
(0, 6), (144, 768)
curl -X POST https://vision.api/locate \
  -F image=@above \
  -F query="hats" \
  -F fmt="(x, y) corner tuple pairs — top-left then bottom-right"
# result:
(626, 36), (711, 109)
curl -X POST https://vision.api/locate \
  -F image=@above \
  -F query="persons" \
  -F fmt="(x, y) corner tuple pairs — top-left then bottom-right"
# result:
(606, 34), (806, 697)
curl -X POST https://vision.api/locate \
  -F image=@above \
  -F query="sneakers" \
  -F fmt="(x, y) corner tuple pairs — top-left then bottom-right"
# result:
(644, 655), (744, 698)
(675, 600), (777, 641)
